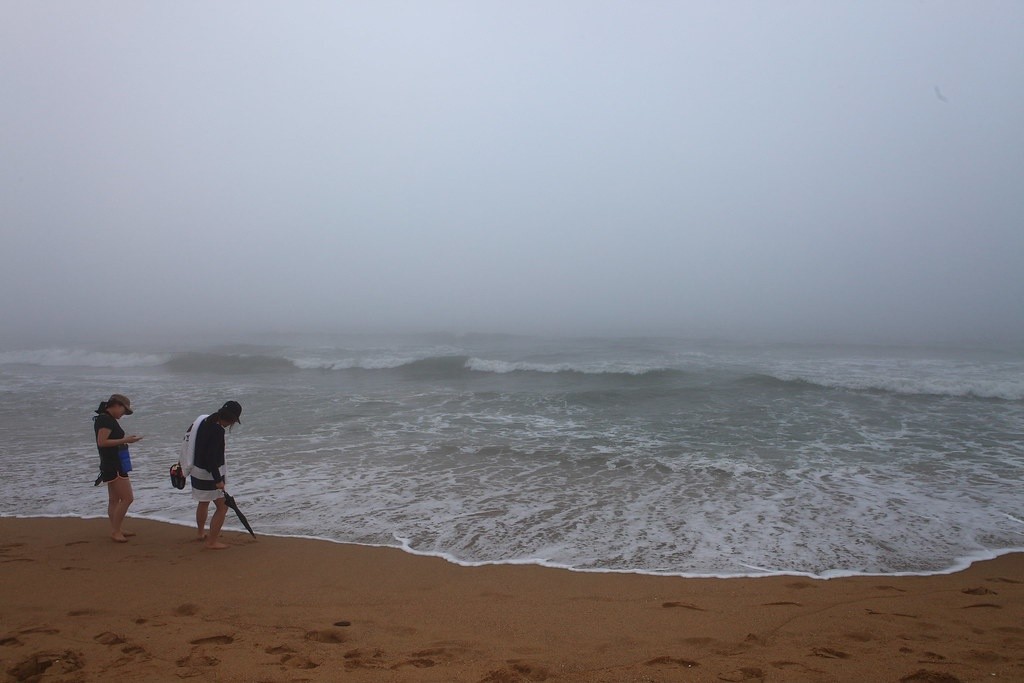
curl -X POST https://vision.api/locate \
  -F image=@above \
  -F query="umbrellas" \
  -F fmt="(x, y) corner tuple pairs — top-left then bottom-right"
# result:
(223, 489), (256, 539)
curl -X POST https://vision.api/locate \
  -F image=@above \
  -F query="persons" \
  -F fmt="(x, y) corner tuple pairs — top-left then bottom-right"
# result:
(177, 401), (242, 550)
(92, 394), (143, 541)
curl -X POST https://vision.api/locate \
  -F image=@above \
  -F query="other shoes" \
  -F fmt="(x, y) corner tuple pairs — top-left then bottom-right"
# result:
(169, 464), (185, 490)
(94, 470), (103, 486)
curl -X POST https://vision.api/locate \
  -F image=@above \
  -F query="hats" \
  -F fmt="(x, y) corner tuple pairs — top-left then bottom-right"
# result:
(221, 401), (242, 425)
(108, 394), (133, 416)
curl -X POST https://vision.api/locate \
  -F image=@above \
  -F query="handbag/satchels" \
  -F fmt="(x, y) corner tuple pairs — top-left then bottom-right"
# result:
(118, 443), (132, 475)
